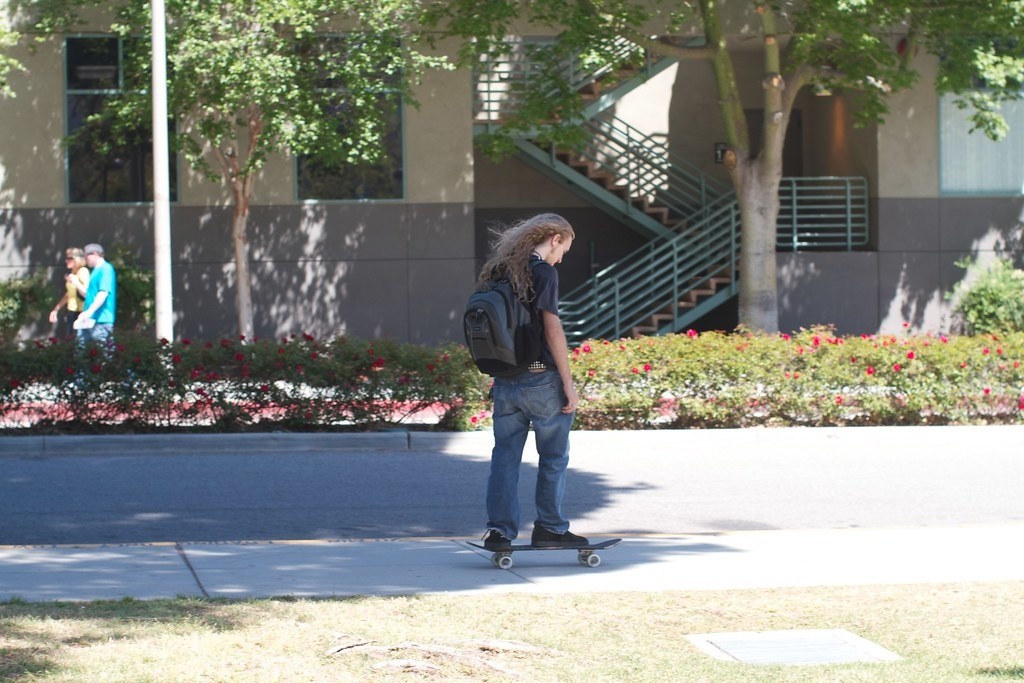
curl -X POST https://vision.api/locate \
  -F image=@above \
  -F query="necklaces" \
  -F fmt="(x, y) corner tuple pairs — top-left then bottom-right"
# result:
(533, 249), (543, 260)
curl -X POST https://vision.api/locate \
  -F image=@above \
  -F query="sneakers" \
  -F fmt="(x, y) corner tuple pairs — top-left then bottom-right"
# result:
(481, 530), (511, 547)
(531, 525), (589, 547)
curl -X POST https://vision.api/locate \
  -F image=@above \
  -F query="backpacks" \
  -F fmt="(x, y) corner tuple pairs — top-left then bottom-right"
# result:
(464, 259), (549, 375)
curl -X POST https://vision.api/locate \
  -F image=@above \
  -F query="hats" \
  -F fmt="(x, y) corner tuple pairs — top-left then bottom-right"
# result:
(78, 244), (103, 257)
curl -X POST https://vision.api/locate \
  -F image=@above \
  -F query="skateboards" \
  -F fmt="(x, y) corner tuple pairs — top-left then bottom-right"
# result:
(464, 534), (628, 571)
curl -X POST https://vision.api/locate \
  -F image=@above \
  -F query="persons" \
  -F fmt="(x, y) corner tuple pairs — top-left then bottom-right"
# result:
(49, 242), (135, 386)
(475, 212), (589, 548)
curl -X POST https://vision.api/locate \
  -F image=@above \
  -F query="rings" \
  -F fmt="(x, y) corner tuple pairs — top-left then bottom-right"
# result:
(572, 402), (577, 408)
